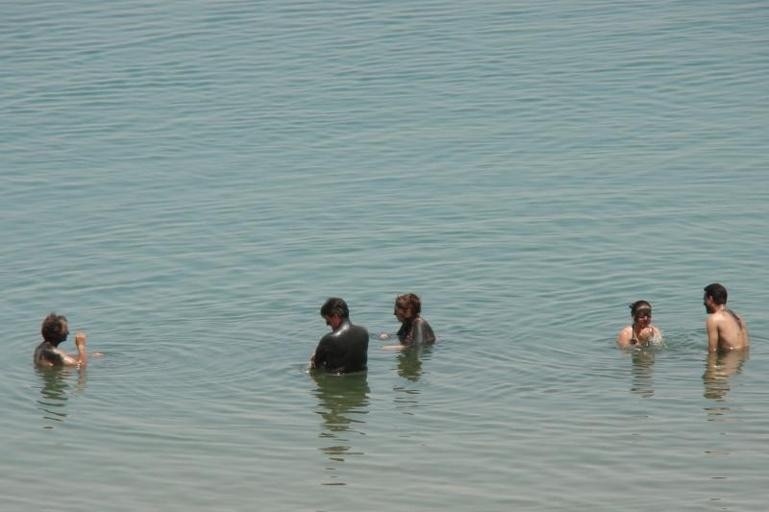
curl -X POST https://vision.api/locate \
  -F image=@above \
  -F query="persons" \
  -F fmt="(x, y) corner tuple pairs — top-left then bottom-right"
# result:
(382, 296), (435, 350)
(703, 284), (749, 350)
(35, 315), (105, 369)
(619, 300), (663, 349)
(311, 298), (372, 374)
